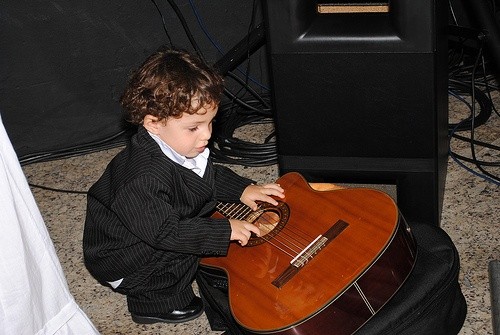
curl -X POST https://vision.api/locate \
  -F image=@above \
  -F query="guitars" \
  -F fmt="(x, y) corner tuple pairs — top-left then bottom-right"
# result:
(196, 172), (421, 335)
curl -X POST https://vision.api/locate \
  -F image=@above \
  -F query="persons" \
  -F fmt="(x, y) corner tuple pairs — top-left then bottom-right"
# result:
(81, 51), (286, 325)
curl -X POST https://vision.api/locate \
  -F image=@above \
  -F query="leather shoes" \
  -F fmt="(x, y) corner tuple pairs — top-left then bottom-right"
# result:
(131, 295), (204, 324)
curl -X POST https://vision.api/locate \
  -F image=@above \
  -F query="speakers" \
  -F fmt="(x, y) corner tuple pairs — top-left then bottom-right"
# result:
(262, 1), (451, 228)
